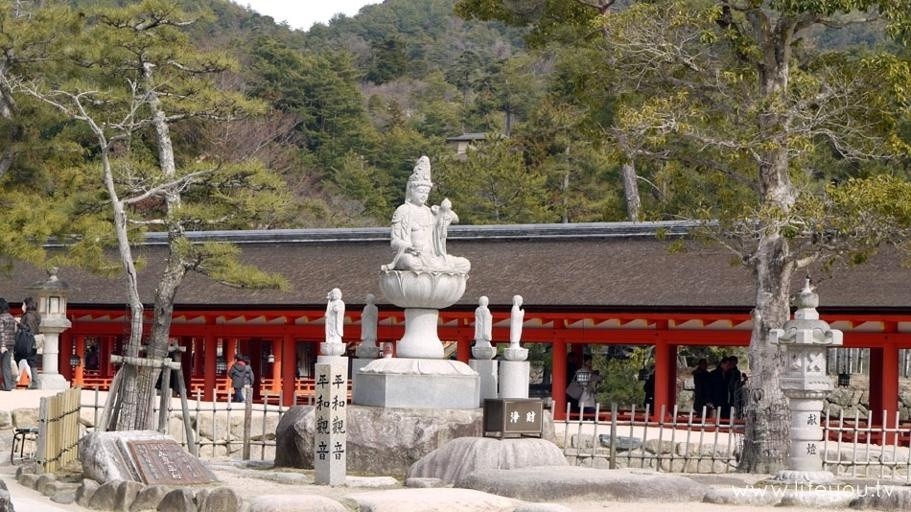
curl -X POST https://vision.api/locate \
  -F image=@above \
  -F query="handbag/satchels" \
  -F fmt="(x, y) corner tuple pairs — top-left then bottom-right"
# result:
(14, 331), (37, 355)
(566, 380), (584, 400)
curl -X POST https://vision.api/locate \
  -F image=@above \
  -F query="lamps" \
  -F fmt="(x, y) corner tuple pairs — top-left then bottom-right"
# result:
(576, 345), (591, 386)
(838, 347), (851, 387)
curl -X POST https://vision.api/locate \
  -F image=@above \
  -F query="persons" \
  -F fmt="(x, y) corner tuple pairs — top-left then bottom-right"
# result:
(381, 155), (471, 274)
(644, 368), (655, 415)
(241, 356), (255, 402)
(474, 295), (492, 347)
(692, 355), (741, 424)
(509, 295), (525, 348)
(324, 288), (345, 343)
(86, 345), (98, 370)
(568, 352), (602, 421)
(361, 294), (378, 348)
(431, 198), (459, 256)
(0, 298), (15, 391)
(228, 354), (246, 403)
(14, 297), (41, 389)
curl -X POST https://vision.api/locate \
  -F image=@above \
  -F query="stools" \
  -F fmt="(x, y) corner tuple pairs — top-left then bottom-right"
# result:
(12, 427), (39, 465)
